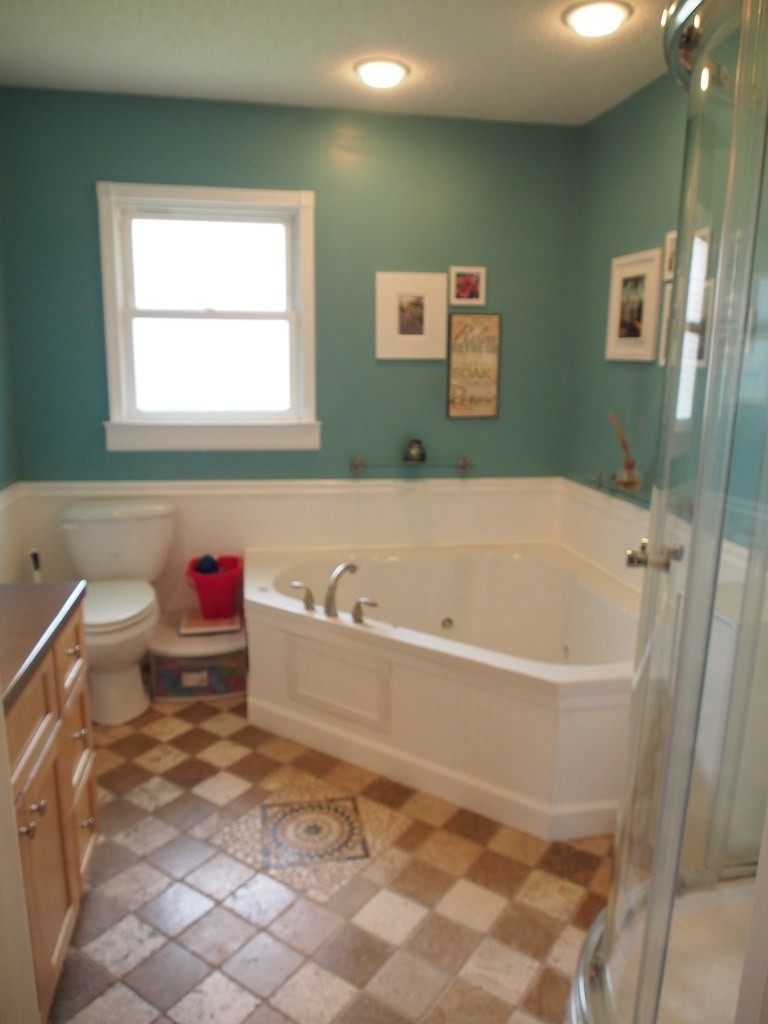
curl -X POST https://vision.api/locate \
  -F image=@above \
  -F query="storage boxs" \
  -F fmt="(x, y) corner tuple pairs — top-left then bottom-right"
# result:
(149, 609), (248, 702)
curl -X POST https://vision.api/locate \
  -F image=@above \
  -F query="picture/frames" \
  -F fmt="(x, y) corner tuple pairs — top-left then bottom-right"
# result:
(662, 227), (712, 281)
(448, 265), (487, 306)
(373, 271), (448, 361)
(658, 278), (717, 369)
(604, 246), (663, 362)
(448, 314), (502, 419)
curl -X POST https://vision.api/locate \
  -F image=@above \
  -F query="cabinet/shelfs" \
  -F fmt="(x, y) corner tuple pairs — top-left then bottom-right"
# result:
(2, 580), (101, 1023)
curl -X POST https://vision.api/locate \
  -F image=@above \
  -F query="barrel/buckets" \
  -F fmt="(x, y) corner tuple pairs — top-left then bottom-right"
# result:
(186, 555), (244, 618)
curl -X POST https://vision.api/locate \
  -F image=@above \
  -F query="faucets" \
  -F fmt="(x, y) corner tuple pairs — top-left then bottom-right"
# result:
(321, 559), (359, 617)
(626, 538), (688, 571)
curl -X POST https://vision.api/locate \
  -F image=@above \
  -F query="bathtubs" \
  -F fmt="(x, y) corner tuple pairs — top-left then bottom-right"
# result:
(237, 544), (720, 848)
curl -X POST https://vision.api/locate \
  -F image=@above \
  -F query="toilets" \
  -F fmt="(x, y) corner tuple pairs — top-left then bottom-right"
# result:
(50, 498), (181, 732)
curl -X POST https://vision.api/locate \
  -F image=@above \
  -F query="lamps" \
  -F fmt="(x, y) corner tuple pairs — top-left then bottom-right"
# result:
(560, 0), (635, 38)
(353, 57), (411, 88)
